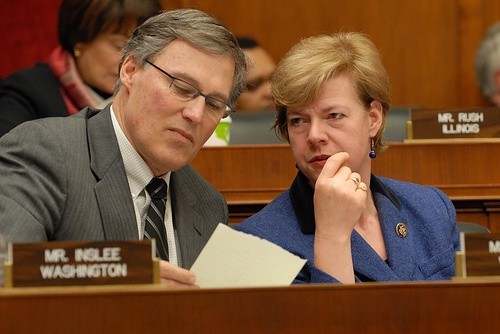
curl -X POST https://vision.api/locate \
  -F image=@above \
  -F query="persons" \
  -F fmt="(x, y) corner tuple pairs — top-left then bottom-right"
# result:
(231, 34), (278, 113)
(0, 0), (165, 140)
(229, 30), (462, 285)
(0, 7), (250, 291)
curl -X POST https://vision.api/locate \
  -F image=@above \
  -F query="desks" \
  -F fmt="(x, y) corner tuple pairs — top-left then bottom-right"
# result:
(182, 139), (500, 239)
(0, 276), (500, 334)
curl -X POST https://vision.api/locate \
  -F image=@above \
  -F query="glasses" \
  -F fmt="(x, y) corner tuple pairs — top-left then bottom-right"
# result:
(145, 58), (235, 120)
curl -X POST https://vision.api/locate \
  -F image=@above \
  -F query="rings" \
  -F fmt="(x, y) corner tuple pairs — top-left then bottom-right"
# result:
(358, 182), (368, 191)
(347, 176), (360, 187)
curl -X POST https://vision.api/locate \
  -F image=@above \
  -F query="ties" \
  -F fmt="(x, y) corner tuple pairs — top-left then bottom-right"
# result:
(143, 178), (169, 263)
